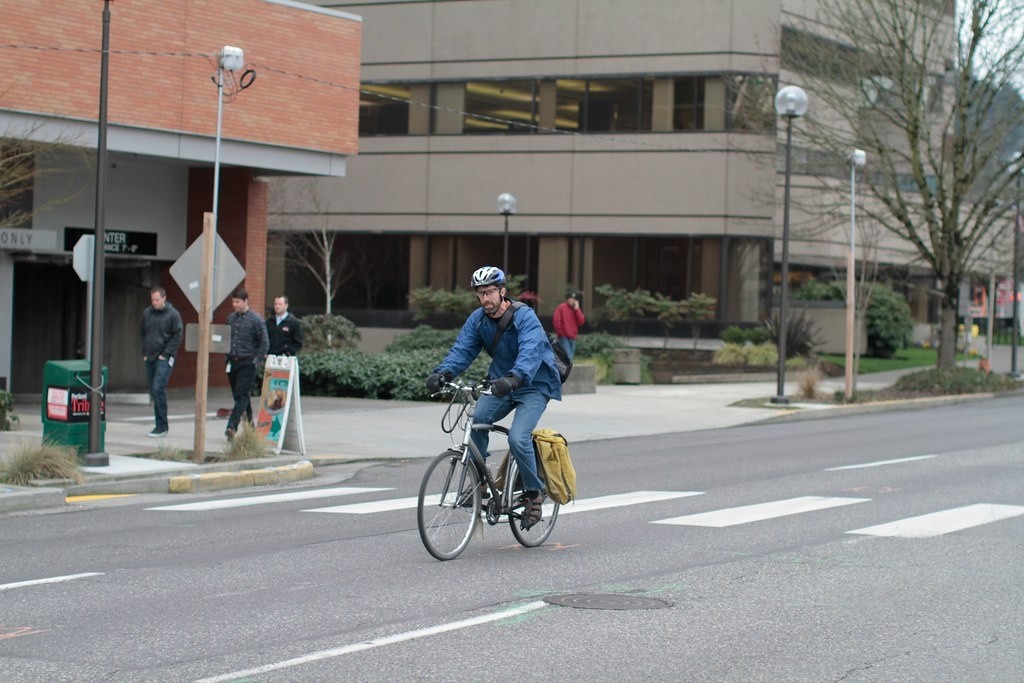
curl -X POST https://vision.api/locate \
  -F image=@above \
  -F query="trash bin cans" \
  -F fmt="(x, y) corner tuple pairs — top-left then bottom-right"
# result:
(613, 348), (641, 384)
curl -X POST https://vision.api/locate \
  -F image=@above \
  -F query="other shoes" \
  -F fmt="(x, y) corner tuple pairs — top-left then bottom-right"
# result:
(226, 428), (234, 442)
(149, 427), (168, 437)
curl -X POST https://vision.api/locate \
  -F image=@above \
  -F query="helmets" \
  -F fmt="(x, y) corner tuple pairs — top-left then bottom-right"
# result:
(471, 267), (506, 288)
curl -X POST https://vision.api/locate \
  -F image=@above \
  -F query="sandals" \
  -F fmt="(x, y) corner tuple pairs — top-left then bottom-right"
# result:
(521, 490), (545, 529)
(460, 483), (488, 505)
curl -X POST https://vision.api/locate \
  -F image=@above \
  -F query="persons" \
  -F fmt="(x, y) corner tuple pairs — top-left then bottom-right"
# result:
(141, 286), (183, 436)
(554, 290), (585, 363)
(427, 266), (562, 528)
(224, 289), (269, 441)
(265, 296), (304, 358)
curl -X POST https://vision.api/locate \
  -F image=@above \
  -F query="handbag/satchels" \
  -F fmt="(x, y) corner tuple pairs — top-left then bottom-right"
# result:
(495, 431), (576, 504)
(546, 334), (572, 384)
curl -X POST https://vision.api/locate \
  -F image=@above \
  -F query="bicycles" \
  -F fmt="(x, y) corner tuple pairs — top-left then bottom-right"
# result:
(418, 377), (561, 562)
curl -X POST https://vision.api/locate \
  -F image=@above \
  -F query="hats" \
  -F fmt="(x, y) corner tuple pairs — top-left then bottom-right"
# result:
(569, 292), (580, 300)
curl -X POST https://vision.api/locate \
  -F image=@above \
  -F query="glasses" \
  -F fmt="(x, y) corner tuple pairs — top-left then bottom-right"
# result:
(477, 285), (504, 297)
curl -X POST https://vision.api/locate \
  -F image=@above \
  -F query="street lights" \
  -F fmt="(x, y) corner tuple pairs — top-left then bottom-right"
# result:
(773, 85), (809, 405)
(498, 193), (517, 277)
(846, 148), (868, 396)
(1007, 152), (1024, 378)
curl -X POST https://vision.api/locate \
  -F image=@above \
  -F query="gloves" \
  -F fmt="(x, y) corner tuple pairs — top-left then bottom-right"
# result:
(490, 376), (519, 399)
(427, 373), (446, 391)
